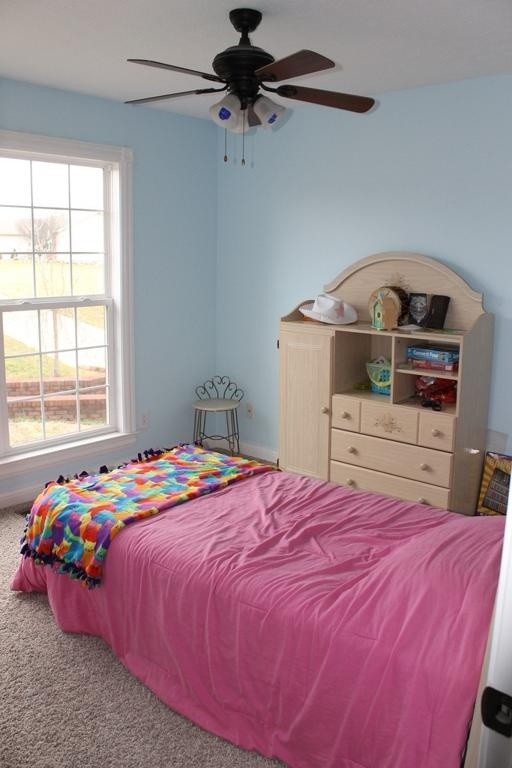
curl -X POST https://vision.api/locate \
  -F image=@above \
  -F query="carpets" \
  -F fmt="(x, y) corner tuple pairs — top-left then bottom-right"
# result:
(1, 510), (292, 767)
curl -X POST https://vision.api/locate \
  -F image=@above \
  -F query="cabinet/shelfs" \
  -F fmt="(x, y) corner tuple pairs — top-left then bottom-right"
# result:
(276, 250), (495, 516)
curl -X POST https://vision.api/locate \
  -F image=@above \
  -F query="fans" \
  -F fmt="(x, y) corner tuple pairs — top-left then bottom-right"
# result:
(124, 7), (375, 114)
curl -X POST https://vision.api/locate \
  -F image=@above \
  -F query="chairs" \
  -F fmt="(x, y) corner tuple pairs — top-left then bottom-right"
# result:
(191, 376), (244, 456)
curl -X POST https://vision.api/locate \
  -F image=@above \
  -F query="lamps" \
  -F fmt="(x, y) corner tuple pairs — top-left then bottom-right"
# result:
(209, 91), (285, 166)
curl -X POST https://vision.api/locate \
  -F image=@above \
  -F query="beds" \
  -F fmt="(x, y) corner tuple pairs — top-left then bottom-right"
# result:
(11, 441), (512, 768)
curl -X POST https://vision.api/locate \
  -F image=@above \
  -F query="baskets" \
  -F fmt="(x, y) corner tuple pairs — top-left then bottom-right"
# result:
(366, 358), (391, 395)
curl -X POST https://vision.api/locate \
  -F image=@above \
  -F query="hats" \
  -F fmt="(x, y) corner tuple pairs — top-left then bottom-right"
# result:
(299, 294), (359, 325)
